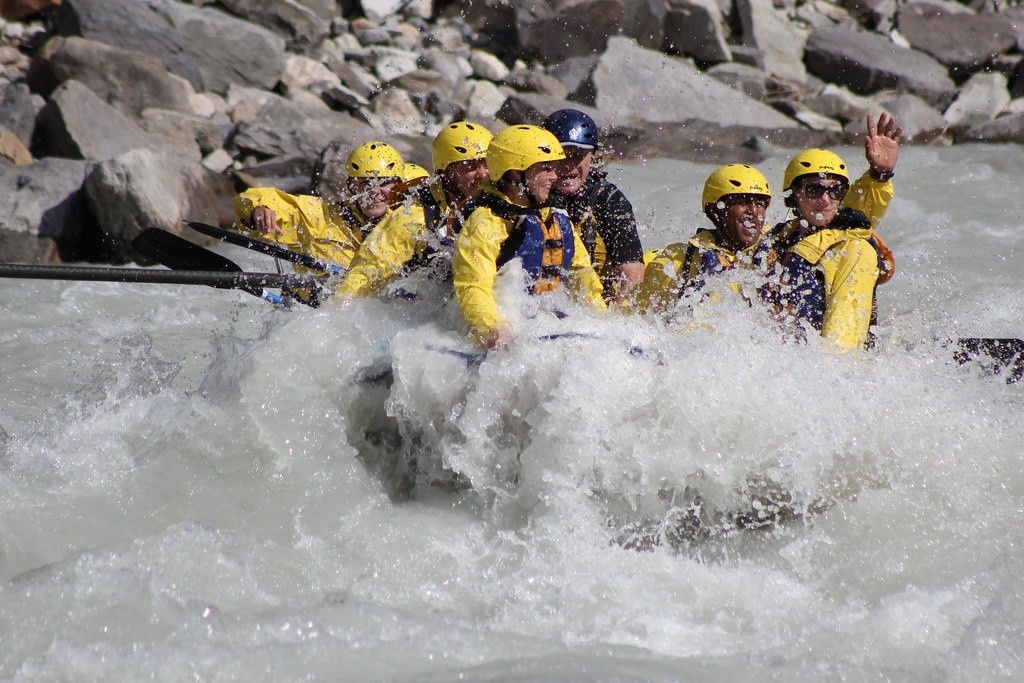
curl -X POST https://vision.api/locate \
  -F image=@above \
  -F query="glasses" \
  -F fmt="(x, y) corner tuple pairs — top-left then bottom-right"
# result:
(801, 182), (847, 200)
(352, 179), (398, 190)
(531, 163), (558, 172)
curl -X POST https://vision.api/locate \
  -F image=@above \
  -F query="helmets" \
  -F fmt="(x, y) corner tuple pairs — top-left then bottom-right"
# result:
(432, 121), (495, 174)
(702, 164), (772, 228)
(403, 162), (431, 183)
(542, 109), (599, 151)
(345, 141), (404, 178)
(486, 124), (567, 182)
(781, 148), (850, 207)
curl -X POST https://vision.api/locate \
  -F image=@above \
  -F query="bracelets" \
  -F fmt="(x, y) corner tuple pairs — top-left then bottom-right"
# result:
(870, 166), (894, 180)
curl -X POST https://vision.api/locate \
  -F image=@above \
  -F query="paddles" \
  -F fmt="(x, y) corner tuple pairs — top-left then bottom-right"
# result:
(182, 219), (348, 273)
(914, 336), (1023, 384)
(129, 227), (284, 307)
(0, 263), (334, 291)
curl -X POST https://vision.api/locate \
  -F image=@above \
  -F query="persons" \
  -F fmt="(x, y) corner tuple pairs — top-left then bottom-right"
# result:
(233, 108), (645, 343)
(637, 111), (902, 360)
